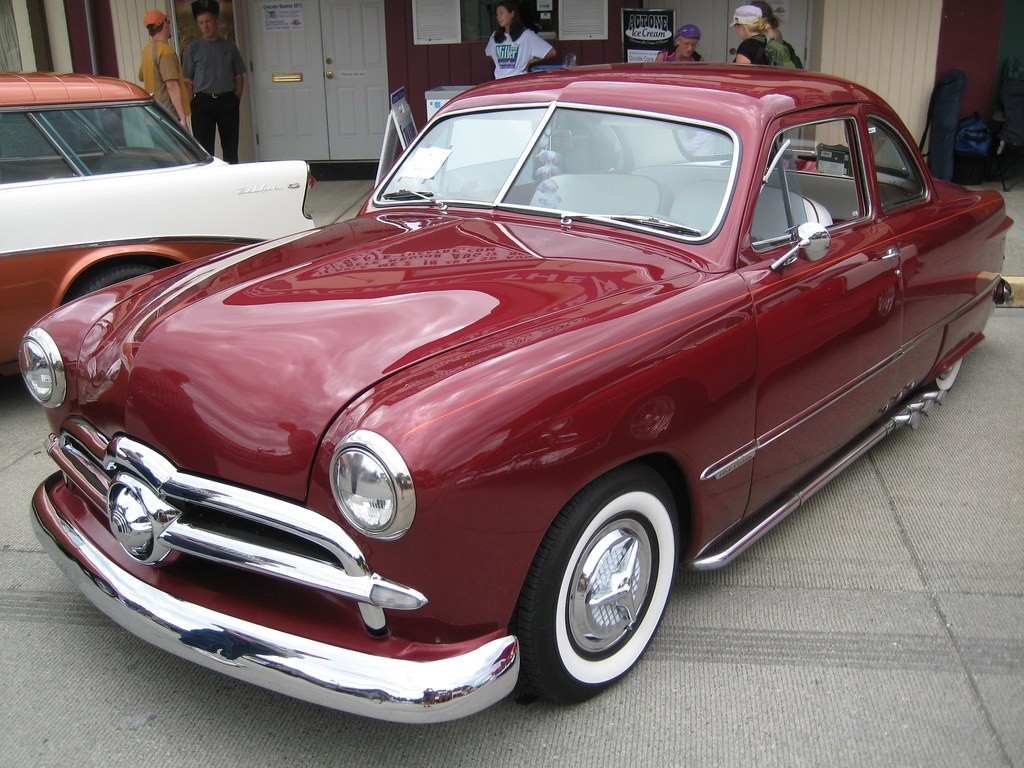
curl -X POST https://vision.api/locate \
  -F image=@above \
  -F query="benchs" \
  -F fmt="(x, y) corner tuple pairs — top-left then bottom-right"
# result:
(632, 165), (859, 225)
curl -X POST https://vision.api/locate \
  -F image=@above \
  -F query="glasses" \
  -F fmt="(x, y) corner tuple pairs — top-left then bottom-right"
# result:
(166, 20), (171, 24)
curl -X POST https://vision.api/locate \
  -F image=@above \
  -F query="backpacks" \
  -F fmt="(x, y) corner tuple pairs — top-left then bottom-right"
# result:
(954, 111), (992, 185)
(750, 36), (796, 68)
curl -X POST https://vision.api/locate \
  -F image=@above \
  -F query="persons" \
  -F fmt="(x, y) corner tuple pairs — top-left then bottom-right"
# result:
(138, 9), (193, 149)
(655, 23), (704, 62)
(484, 0), (556, 80)
(182, 0), (247, 164)
(729, 0), (802, 68)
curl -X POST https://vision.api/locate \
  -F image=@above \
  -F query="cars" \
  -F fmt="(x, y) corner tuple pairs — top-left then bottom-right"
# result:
(19, 63), (1015, 724)
(0, 71), (316, 378)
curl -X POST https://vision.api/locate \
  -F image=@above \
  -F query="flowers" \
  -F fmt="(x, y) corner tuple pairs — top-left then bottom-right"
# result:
(535, 134), (563, 209)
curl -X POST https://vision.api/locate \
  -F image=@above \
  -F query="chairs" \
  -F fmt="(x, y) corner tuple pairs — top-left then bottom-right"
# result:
(529, 173), (662, 215)
(668, 179), (833, 243)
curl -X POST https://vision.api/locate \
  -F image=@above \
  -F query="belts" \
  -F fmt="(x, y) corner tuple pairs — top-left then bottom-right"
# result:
(196, 91), (234, 100)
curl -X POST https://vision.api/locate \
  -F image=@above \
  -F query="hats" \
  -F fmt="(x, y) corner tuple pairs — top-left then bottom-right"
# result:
(675, 24), (701, 40)
(729, 5), (762, 26)
(144, 10), (166, 31)
